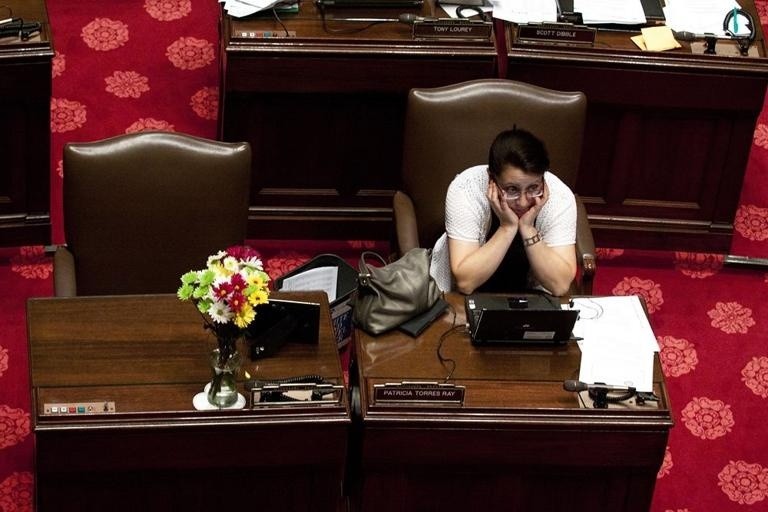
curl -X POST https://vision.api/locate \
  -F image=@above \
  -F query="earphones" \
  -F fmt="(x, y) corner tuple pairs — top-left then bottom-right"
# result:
(568, 300), (574, 308)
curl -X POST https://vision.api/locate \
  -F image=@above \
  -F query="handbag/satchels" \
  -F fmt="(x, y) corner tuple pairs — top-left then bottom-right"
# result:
(351, 248), (442, 337)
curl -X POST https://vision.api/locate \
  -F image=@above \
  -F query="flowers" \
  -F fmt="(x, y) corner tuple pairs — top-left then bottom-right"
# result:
(177, 246), (272, 399)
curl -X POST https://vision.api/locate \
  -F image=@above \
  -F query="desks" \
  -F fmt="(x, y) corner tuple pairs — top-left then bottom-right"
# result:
(355, 293), (674, 512)
(216, 0), (498, 241)
(503, 0), (768, 254)
(26, 290), (352, 512)
(0, 0), (56, 246)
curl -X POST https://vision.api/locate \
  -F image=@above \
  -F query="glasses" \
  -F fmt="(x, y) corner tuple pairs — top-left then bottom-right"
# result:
(495, 177), (544, 200)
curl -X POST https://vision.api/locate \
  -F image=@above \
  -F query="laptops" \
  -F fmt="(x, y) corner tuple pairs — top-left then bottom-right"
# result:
(465, 293), (581, 348)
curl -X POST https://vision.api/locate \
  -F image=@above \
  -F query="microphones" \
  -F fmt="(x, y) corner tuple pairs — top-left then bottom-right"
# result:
(563, 379), (636, 393)
(0, 16), (22, 26)
(272, 0), (418, 41)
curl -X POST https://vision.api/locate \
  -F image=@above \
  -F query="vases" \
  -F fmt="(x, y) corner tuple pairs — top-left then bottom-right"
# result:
(208, 348), (242, 408)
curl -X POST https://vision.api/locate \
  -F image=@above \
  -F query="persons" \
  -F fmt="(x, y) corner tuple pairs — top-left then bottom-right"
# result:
(430, 128), (578, 297)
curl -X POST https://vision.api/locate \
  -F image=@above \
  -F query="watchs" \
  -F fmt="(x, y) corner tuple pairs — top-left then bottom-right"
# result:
(519, 232), (543, 247)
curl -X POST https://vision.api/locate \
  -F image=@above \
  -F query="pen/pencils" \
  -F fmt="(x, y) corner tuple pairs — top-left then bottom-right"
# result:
(733, 6), (739, 33)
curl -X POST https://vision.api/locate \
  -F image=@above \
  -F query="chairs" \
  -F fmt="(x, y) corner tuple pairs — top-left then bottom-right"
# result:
(393, 78), (596, 296)
(52, 130), (252, 297)
